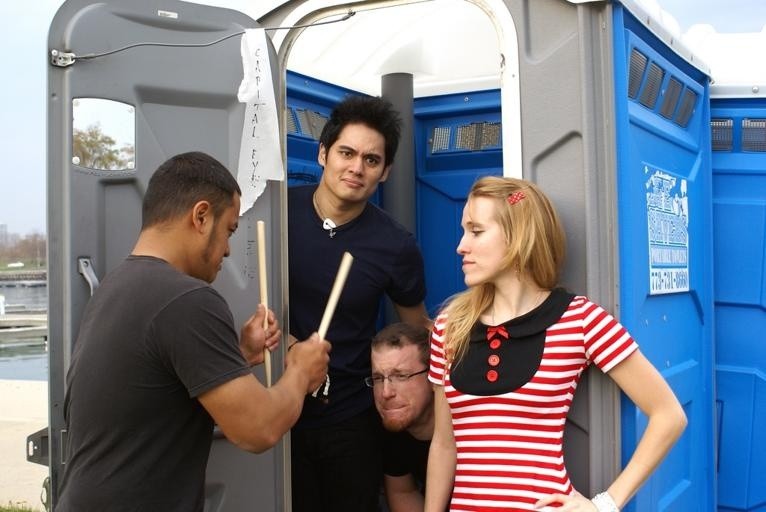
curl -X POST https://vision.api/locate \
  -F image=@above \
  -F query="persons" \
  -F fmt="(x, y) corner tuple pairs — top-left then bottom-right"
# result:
(288, 95), (428, 512)
(53, 151), (330, 512)
(371, 322), (434, 511)
(424, 176), (688, 512)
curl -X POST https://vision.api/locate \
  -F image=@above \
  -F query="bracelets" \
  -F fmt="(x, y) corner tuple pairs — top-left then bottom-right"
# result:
(593, 492), (619, 512)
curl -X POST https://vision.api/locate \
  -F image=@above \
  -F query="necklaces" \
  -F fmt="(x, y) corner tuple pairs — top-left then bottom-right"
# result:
(313, 193), (364, 234)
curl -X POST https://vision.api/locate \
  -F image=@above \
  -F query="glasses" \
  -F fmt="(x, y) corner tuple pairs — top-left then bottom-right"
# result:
(364, 367), (429, 388)
